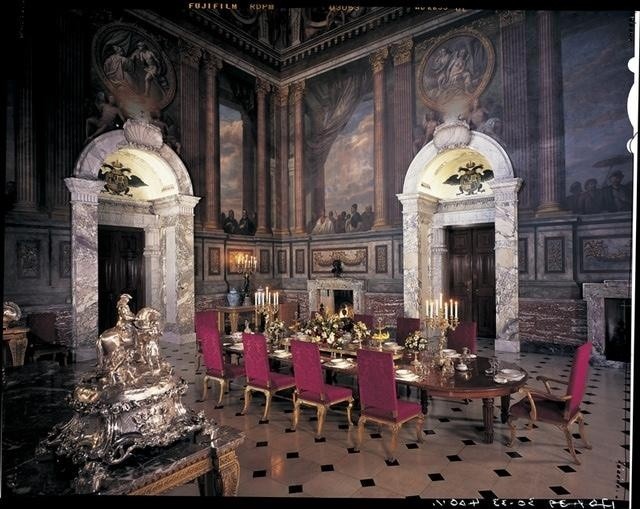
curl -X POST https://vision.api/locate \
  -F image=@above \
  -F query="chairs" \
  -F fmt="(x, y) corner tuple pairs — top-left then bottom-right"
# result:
(27, 313), (69, 368)
(508, 342), (593, 465)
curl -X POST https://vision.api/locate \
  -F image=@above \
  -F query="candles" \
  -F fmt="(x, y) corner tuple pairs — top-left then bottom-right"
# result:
(252, 283), (280, 312)
(420, 291), (462, 327)
(235, 254), (257, 269)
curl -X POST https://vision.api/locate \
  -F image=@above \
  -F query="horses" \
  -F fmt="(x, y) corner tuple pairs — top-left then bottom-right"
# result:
(96, 307), (161, 368)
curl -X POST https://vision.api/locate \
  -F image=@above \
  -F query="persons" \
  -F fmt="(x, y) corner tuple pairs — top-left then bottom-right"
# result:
(578, 179), (607, 214)
(306, 203), (374, 233)
(114, 293), (140, 344)
(603, 170), (632, 212)
(433, 48), (450, 97)
(82, 92), (126, 144)
(237, 208), (257, 236)
(222, 208), (240, 234)
(133, 41), (168, 98)
(444, 49), (472, 96)
(103, 44), (135, 84)
(565, 180), (582, 210)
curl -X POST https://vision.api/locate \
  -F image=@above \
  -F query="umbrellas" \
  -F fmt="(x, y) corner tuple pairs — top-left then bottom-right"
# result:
(589, 153), (632, 185)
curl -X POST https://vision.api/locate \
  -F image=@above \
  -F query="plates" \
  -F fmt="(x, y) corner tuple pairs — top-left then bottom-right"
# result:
(221, 328), (524, 389)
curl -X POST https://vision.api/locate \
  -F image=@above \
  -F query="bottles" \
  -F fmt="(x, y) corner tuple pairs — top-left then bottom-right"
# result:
(227, 287), (240, 306)
(256, 285), (266, 305)
